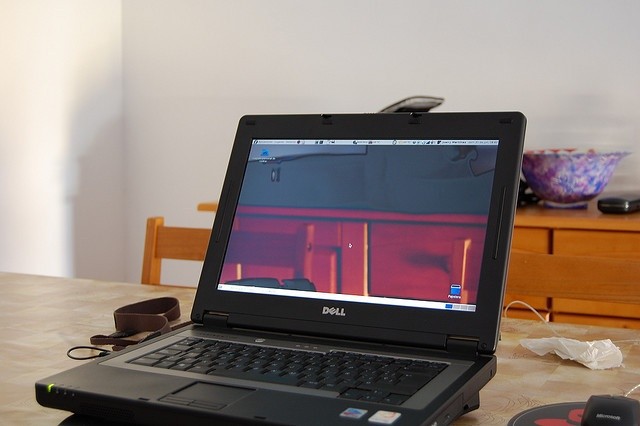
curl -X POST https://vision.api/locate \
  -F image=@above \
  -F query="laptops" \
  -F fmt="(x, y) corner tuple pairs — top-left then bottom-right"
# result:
(35, 111), (526, 426)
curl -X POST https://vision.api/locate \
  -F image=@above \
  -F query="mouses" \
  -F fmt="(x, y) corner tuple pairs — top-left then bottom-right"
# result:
(582, 395), (637, 426)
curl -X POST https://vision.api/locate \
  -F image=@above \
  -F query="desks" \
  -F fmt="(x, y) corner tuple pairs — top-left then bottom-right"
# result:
(1, 271), (640, 425)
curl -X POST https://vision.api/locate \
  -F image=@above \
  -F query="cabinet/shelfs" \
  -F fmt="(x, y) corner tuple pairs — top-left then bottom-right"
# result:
(502, 190), (640, 329)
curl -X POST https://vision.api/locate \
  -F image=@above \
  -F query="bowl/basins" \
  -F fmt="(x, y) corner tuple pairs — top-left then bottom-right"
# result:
(521, 147), (630, 209)
